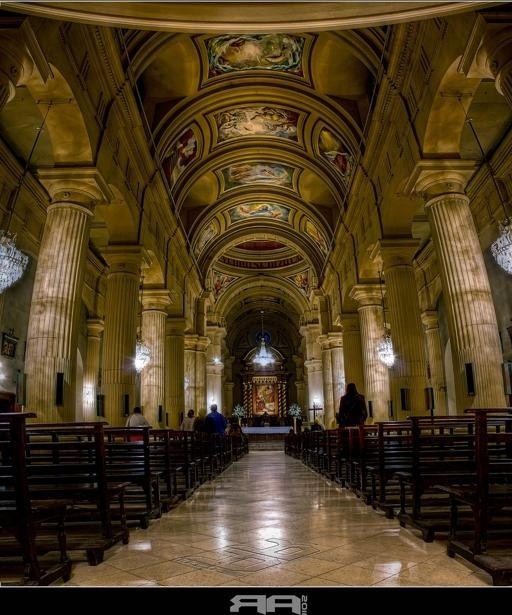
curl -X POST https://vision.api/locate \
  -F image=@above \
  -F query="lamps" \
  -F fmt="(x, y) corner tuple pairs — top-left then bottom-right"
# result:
(455, 91), (512, 276)
(136, 276), (152, 375)
(0, 98), (55, 295)
(374, 271), (395, 369)
(252, 310), (277, 368)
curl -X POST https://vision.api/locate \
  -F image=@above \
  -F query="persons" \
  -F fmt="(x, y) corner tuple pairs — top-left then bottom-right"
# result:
(126, 407), (150, 442)
(303, 418), (322, 432)
(256, 386), (274, 412)
(339, 383), (367, 427)
(180, 404), (246, 440)
(288, 429), (296, 436)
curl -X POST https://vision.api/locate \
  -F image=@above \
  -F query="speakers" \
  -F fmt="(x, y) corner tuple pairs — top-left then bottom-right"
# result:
(97, 395), (104, 416)
(368, 401), (373, 417)
(16, 372), (24, 405)
(123, 394), (129, 416)
(504, 362), (512, 395)
(159, 405), (162, 422)
(166, 413), (168, 426)
(55, 373), (64, 405)
(389, 400), (393, 416)
(465, 363), (476, 395)
(181, 412), (183, 424)
(427, 388), (434, 409)
(400, 388), (408, 410)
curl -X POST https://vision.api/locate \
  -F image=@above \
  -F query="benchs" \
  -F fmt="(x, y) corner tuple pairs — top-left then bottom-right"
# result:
(283, 405), (511, 588)
(1, 411), (249, 587)
